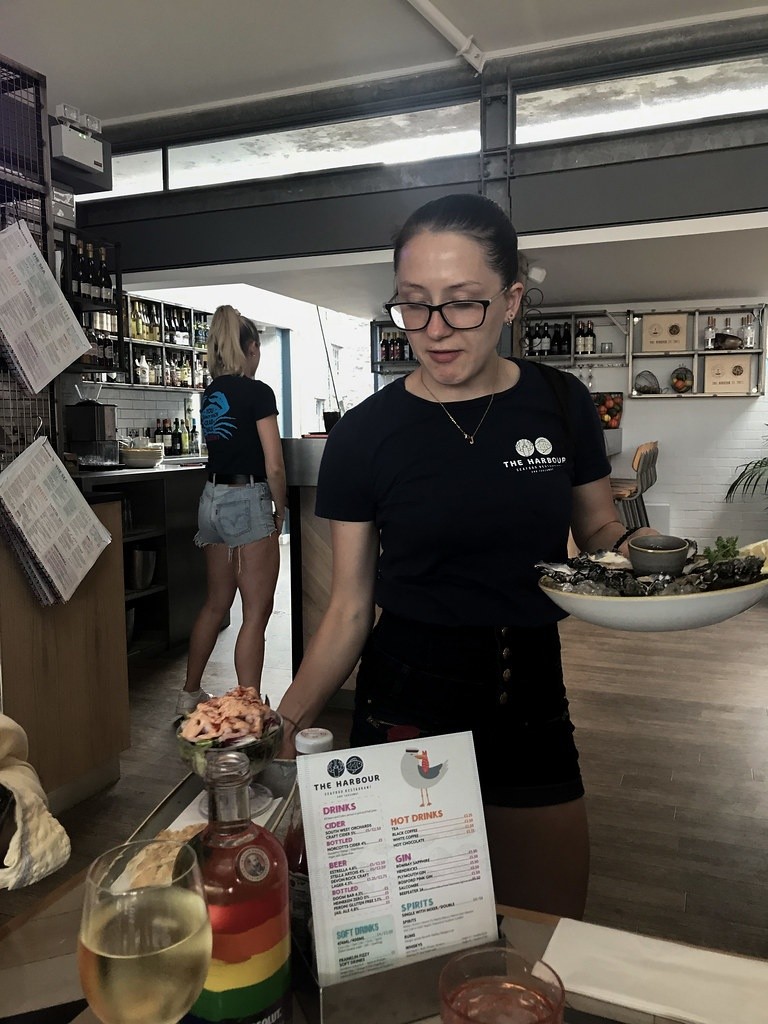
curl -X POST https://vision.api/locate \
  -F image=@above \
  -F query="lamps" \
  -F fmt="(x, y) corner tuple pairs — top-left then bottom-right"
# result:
(52, 103), (105, 175)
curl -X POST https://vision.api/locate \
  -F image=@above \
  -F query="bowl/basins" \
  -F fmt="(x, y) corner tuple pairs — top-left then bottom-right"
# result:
(628, 534), (690, 574)
(74, 383), (102, 401)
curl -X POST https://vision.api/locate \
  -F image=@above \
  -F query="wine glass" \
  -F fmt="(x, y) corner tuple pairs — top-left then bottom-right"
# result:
(77, 837), (212, 1024)
(577, 364), (596, 391)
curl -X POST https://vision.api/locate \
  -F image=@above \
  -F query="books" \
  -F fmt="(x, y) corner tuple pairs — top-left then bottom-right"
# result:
(0, 436), (113, 609)
(0, 219), (92, 397)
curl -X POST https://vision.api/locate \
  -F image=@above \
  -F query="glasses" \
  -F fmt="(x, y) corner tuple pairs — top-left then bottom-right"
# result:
(383, 288), (508, 331)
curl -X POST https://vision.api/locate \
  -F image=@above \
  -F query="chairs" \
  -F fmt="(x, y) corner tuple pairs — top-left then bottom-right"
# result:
(608, 441), (660, 528)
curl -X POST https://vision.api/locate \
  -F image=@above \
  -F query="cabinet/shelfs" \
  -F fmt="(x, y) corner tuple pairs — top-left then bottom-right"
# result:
(371, 321), (420, 374)
(520, 311), (628, 359)
(628, 304), (768, 399)
(87, 483), (169, 657)
(76, 292), (210, 394)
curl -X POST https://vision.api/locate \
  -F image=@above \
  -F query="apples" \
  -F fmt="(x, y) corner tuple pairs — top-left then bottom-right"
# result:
(673, 374), (692, 390)
(596, 395), (622, 428)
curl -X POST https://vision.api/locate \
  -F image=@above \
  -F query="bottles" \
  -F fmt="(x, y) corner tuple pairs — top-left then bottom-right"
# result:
(703, 314), (755, 350)
(523, 321), (597, 355)
(68, 241), (212, 388)
(282, 727), (335, 936)
(146, 419), (200, 455)
(381, 329), (412, 361)
(182, 750), (291, 1024)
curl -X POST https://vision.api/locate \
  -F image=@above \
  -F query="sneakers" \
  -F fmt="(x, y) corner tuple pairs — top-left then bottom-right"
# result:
(175, 689), (213, 715)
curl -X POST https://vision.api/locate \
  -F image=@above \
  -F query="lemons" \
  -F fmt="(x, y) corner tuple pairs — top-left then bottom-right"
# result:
(735, 539), (768, 574)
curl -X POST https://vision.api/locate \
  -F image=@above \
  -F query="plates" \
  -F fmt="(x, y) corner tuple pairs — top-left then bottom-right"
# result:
(119, 449), (164, 467)
(538, 567), (767, 632)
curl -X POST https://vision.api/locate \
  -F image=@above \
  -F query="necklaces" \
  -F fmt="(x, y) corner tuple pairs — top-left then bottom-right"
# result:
(420, 357), (499, 444)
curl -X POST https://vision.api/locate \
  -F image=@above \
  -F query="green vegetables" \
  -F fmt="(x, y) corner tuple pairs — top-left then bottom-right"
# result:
(703, 536), (740, 566)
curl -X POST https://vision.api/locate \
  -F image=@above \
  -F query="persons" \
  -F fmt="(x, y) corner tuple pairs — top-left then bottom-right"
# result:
(276, 194), (663, 920)
(175, 305), (288, 714)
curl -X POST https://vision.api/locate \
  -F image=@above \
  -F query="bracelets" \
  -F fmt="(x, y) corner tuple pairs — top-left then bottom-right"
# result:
(611, 526), (640, 552)
(274, 511), (286, 521)
(281, 715), (301, 732)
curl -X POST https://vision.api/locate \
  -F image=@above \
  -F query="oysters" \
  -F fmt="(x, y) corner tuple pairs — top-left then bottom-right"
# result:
(532, 538), (767, 597)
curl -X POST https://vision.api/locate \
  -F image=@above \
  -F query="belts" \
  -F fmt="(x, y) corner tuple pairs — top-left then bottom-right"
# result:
(209, 472), (264, 485)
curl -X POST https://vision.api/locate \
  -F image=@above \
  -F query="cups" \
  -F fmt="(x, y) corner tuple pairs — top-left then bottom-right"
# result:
(439, 947), (567, 1023)
(601, 342), (612, 353)
